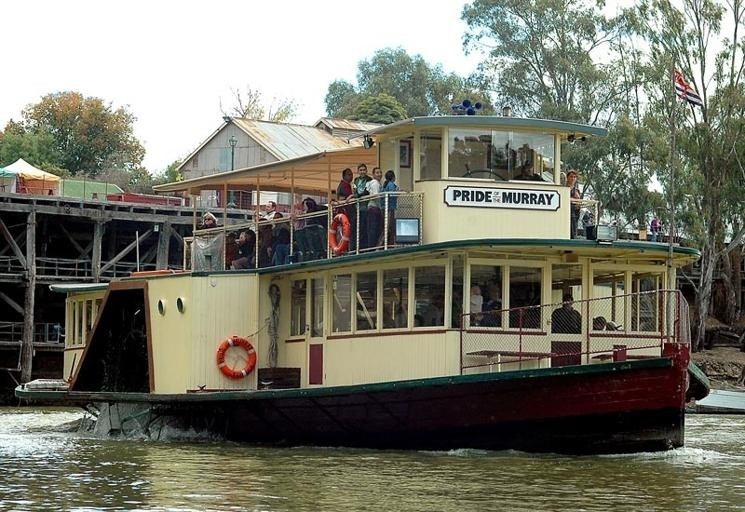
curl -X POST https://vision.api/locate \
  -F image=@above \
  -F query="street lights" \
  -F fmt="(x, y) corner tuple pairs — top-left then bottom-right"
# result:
(229, 133), (239, 208)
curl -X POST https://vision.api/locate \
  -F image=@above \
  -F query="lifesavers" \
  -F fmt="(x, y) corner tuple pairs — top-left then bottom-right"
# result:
(216, 336), (257, 379)
(327, 214), (351, 253)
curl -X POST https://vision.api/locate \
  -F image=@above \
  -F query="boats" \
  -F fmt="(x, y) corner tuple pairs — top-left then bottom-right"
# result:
(14, 119), (713, 455)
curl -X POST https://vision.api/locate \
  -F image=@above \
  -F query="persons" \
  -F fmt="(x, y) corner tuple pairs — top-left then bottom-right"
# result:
(565, 169), (584, 240)
(349, 163), (373, 255)
(375, 169), (411, 254)
(190, 197), (327, 272)
(413, 277), (623, 333)
(365, 166), (386, 253)
(649, 215), (661, 242)
(333, 167), (353, 254)
(512, 159), (548, 182)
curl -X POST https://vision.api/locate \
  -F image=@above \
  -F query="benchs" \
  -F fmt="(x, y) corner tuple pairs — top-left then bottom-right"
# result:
(467, 350), (559, 372)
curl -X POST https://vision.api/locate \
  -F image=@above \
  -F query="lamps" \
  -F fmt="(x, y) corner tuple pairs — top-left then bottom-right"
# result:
(395, 218), (421, 246)
(363, 137), (374, 149)
(587, 224), (619, 245)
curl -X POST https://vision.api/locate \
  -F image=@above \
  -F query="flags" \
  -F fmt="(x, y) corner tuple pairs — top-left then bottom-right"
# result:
(674, 67), (703, 106)
